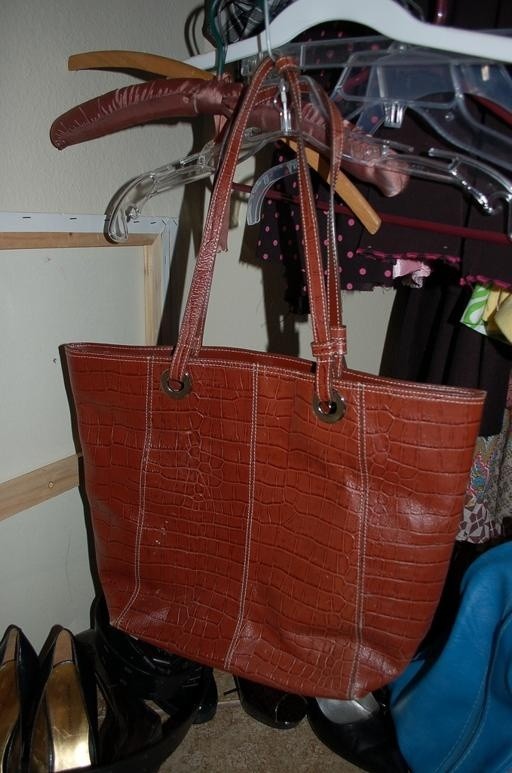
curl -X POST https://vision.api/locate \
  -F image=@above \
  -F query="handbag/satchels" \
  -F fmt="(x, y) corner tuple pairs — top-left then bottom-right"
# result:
(391, 536), (512, 773)
(48, 342), (488, 702)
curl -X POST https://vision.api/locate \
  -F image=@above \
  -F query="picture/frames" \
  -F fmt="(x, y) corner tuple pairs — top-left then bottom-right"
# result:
(0, 214), (181, 524)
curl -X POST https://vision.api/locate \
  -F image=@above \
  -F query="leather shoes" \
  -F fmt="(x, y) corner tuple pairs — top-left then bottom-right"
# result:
(27, 624), (99, 772)
(307, 688), (412, 773)
(233, 676), (305, 729)
(0, 623), (40, 773)
(90, 592), (223, 773)
